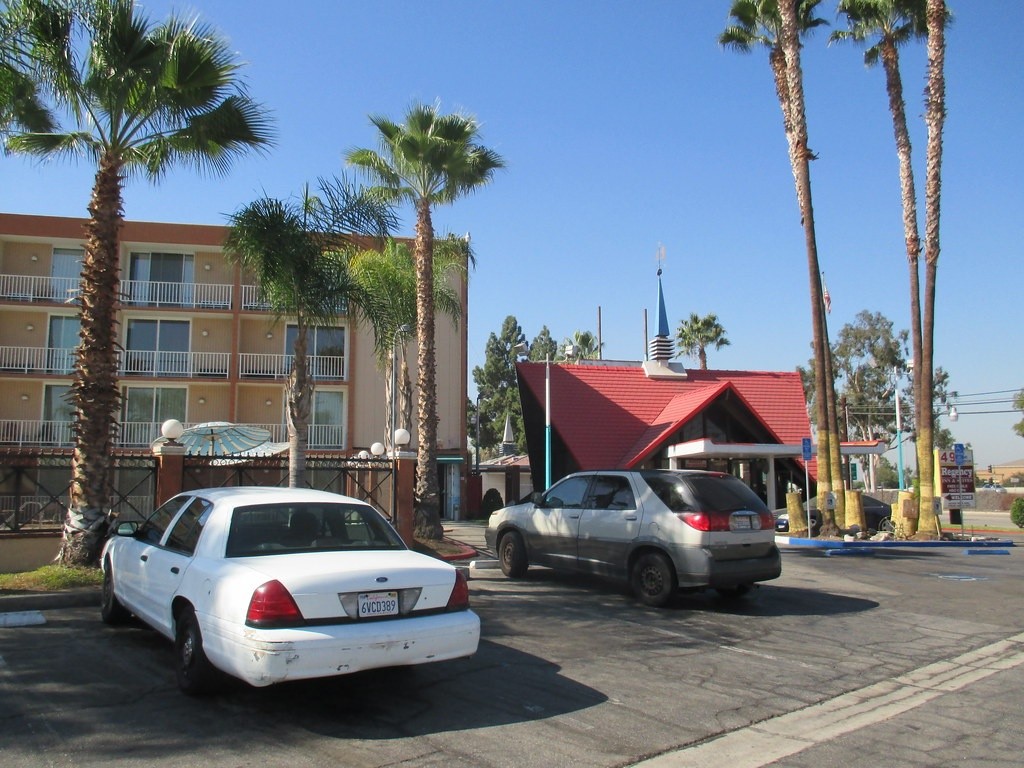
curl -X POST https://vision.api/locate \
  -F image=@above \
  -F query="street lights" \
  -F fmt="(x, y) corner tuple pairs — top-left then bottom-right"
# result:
(475, 390), (487, 519)
(866, 354), (914, 490)
(392, 324), (411, 529)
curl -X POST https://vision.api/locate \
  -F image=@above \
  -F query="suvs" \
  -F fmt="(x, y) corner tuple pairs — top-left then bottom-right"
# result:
(485, 469), (783, 608)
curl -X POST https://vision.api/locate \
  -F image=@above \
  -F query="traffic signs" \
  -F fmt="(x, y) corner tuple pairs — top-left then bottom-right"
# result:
(938, 447), (977, 511)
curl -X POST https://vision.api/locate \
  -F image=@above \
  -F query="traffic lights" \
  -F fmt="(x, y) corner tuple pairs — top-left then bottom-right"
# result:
(988, 465), (993, 474)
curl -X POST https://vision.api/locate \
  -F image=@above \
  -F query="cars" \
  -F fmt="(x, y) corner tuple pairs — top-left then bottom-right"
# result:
(100, 485), (482, 695)
(983, 483), (1007, 493)
(774, 494), (894, 537)
